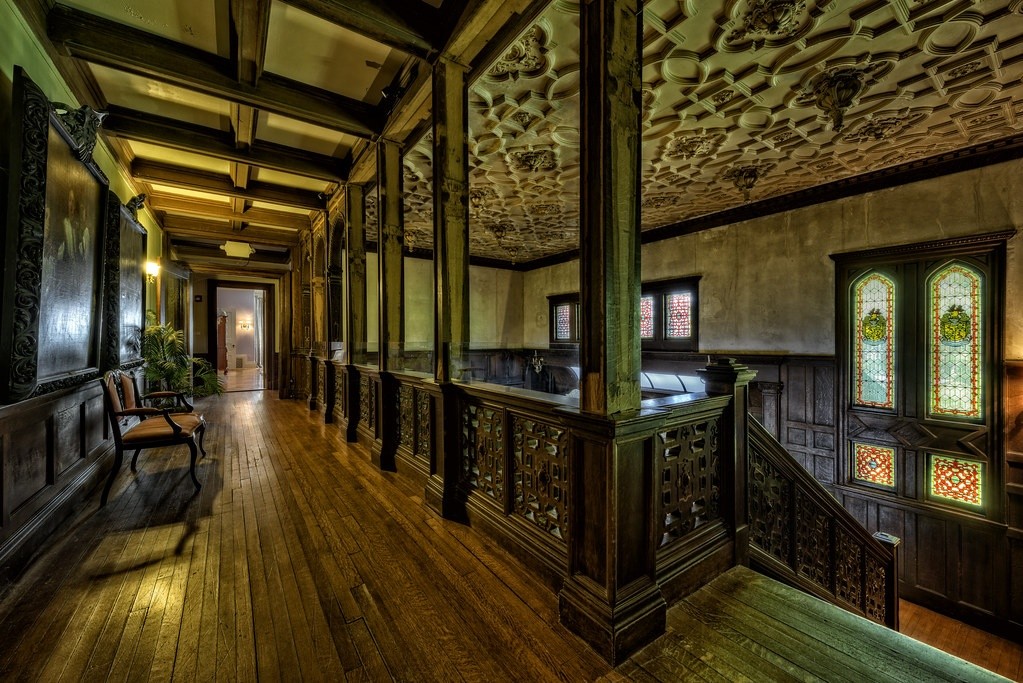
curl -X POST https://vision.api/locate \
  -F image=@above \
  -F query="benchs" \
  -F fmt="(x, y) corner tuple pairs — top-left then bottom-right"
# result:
(100, 369), (208, 506)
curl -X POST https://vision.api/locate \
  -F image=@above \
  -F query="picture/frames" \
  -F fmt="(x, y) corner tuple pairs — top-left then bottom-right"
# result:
(0, 65), (149, 402)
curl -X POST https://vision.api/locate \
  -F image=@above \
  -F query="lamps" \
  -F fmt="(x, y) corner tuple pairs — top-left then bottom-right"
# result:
(147, 262), (161, 284)
(239, 319), (252, 330)
